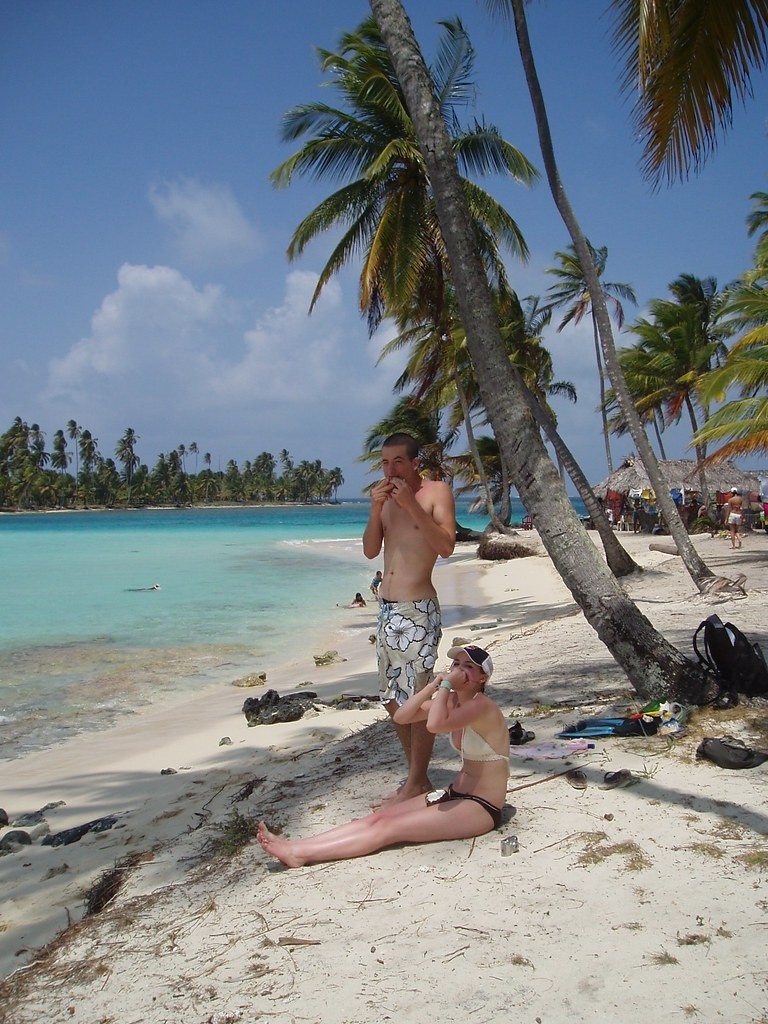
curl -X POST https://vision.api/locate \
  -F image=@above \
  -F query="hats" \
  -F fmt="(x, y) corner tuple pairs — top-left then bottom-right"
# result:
(731, 487), (738, 493)
(447, 644), (493, 678)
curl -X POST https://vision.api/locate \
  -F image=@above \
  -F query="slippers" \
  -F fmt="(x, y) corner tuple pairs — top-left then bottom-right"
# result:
(598, 769), (631, 790)
(566, 771), (588, 789)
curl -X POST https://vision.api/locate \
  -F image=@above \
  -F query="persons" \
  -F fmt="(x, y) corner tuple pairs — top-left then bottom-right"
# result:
(725, 487), (744, 549)
(256, 645), (509, 868)
(633, 498), (643, 533)
(363, 434), (455, 811)
(370, 571), (383, 594)
(351, 593), (366, 606)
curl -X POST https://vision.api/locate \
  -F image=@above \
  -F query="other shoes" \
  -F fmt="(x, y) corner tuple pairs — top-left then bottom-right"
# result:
(508, 720), (535, 745)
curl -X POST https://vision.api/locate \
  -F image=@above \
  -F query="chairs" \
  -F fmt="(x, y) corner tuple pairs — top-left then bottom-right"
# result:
(523, 516), (533, 529)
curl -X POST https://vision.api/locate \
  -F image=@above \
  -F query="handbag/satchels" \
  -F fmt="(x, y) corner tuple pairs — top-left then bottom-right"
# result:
(696, 734), (768, 770)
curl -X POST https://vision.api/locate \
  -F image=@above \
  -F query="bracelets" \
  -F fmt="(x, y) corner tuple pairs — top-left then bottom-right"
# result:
(441, 680), (452, 691)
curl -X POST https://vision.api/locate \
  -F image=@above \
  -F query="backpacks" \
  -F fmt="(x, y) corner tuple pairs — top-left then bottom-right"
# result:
(693, 614), (768, 709)
